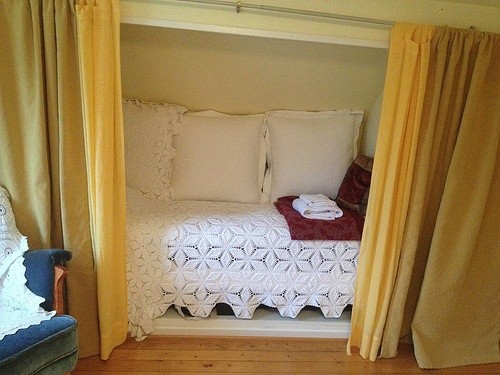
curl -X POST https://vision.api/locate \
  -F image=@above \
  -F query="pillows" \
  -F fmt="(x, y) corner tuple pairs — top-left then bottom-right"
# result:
(121, 96), (188, 198)
(267, 105), (364, 202)
(171, 110), (267, 205)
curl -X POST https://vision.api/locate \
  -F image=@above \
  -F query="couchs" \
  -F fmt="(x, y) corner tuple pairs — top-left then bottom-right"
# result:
(117, 155), (376, 319)
(0, 186), (78, 375)
